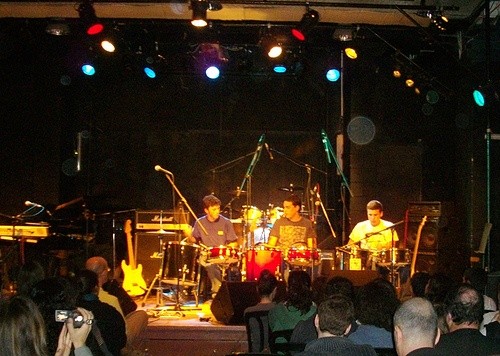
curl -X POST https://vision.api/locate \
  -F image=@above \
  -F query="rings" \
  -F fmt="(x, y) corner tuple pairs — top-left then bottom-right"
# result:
(83, 319), (89, 322)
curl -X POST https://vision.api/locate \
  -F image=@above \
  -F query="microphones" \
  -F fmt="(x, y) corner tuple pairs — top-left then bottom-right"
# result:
(309, 186), (317, 201)
(25, 201), (42, 208)
(265, 142), (273, 159)
(155, 165), (173, 175)
(322, 139), (328, 152)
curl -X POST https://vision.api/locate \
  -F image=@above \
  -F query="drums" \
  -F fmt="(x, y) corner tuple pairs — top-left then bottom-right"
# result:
(161, 241), (201, 286)
(242, 245), (283, 284)
(309, 252), (344, 283)
(286, 247), (322, 266)
(375, 248), (410, 267)
(241, 205), (262, 223)
(341, 247), (373, 274)
(204, 246), (240, 263)
(265, 206), (284, 223)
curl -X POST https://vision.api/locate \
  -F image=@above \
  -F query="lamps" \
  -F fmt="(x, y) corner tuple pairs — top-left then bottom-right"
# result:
(72, 0), (500, 108)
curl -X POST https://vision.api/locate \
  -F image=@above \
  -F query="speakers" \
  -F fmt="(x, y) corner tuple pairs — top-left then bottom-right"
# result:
(326, 270), (381, 286)
(129, 232), (182, 290)
(405, 215), (456, 277)
(210, 281), (261, 325)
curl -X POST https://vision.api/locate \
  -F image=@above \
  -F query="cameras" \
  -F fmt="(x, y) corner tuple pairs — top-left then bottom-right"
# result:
(55, 310), (84, 328)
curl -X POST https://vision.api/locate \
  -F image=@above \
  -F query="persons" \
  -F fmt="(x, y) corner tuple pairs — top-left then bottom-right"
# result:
(0, 238), (149, 356)
(268, 194), (318, 291)
(392, 268), (500, 356)
(187, 194), (238, 299)
(348, 200), (399, 269)
(244, 264), (402, 356)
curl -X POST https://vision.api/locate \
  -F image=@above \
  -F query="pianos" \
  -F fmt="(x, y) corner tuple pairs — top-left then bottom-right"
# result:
(0, 225), (51, 293)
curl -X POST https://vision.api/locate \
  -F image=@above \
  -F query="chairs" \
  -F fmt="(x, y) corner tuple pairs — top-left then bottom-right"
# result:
(244, 309), (397, 356)
(471, 222), (492, 269)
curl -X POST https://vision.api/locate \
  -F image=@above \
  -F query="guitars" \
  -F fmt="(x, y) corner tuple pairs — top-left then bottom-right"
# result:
(121, 220), (147, 296)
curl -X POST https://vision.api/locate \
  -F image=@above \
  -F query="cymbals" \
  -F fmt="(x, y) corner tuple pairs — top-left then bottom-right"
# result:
(278, 185), (304, 192)
(224, 189), (247, 194)
(299, 209), (322, 217)
